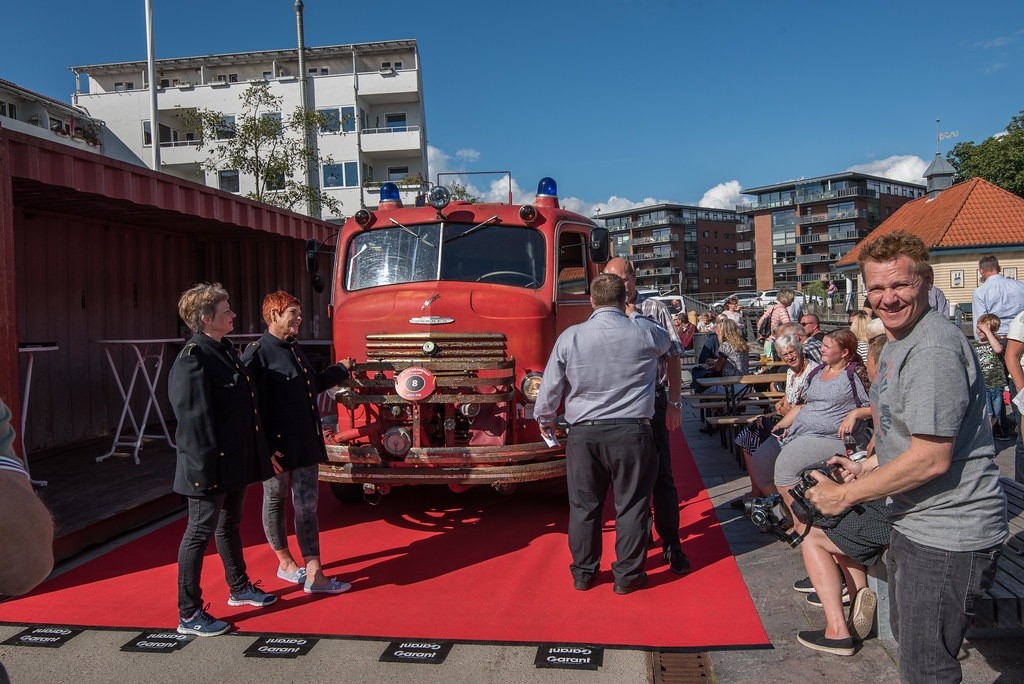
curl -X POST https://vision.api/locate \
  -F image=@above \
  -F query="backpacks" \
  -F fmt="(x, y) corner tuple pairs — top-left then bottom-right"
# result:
(758, 306), (781, 337)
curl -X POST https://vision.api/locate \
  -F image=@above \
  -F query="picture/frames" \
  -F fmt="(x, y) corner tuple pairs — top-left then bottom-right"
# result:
(950, 270), (964, 289)
(1002, 267), (1017, 280)
(976, 268), (986, 286)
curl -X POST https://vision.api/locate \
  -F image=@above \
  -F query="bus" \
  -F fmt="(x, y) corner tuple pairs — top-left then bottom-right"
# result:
(318, 169), (617, 505)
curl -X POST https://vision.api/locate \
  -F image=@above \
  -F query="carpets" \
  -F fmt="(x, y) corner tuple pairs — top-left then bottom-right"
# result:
(0, 424), (778, 653)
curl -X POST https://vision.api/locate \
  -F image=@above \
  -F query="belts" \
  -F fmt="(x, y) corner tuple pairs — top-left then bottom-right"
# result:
(575, 419), (651, 426)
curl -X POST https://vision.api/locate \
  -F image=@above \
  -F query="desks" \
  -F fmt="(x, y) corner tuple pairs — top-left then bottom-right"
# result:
(224, 332), (264, 359)
(297, 340), (333, 346)
(696, 373), (786, 454)
(18, 344), (61, 487)
(681, 360), (787, 423)
(91, 337), (185, 465)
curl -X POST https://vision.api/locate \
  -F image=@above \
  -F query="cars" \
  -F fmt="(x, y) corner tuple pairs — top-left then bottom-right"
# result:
(711, 292), (758, 314)
(757, 290), (826, 308)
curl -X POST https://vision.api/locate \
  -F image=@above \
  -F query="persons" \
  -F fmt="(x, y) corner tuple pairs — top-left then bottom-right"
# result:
(805, 233), (1012, 684)
(533, 272), (671, 594)
(753, 288), (826, 413)
(167, 285), (277, 638)
(698, 298), (750, 432)
(1004, 310), (1024, 485)
(973, 314), (1005, 426)
(928, 287), (950, 319)
(851, 296), (885, 364)
(0, 397), (54, 596)
(241, 290), (356, 593)
(673, 313), (697, 350)
(601, 257), (690, 572)
(735, 330), (892, 656)
(826, 279), (836, 309)
(972, 256), (1024, 440)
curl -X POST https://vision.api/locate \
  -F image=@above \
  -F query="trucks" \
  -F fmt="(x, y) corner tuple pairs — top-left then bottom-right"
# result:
(637, 289), (687, 318)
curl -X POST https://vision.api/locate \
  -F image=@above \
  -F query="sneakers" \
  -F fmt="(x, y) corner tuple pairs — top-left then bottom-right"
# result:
(793, 577), (816, 593)
(304, 577), (351, 593)
(663, 547), (691, 573)
(805, 584), (850, 607)
(227, 580), (279, 606)
(277, 566), (307, 583)
(177, 603), (230, 636)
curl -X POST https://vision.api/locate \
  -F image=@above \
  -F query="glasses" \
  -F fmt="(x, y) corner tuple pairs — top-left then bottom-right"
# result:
(800, 322), (813, 326)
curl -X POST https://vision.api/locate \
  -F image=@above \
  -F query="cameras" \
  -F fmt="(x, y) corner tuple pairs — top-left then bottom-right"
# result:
(789, 463), (845, 515)
(730, 493), (782, 532)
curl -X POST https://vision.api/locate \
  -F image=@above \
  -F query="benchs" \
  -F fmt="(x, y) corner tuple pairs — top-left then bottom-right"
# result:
(865, 475), (1024, 642)
(680, 384), (786, 472)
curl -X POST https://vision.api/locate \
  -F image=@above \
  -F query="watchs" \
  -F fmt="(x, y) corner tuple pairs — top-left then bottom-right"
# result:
(670, 401), (682, 409)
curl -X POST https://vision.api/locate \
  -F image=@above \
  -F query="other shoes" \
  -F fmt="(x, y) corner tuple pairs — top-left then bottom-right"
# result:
(573, 580), (592, 591)
(994, 435), (1010, 440)
(847, 587), (876, 640)
(613, 572), (648, 594)
(796, 629), (855, 656)
